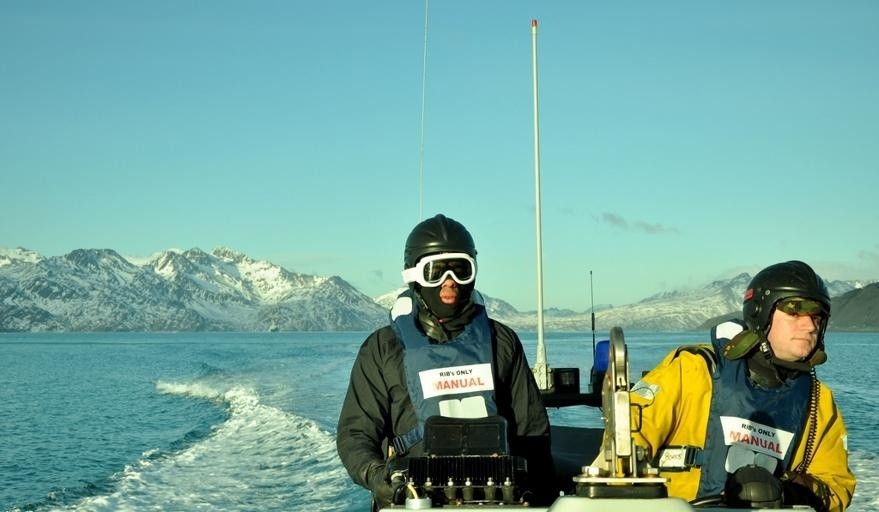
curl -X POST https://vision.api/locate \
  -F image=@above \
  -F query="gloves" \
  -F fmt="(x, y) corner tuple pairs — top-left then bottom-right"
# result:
(367, 461), (401, 510)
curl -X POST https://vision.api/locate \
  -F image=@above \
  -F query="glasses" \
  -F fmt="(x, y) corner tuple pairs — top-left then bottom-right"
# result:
(778, 298), (827, 318)
(402, 253), (478, 288)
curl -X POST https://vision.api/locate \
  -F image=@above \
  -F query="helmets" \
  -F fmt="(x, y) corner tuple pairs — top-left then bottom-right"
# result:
(743, 261), (830, 343)
(403, 215), (477, 292)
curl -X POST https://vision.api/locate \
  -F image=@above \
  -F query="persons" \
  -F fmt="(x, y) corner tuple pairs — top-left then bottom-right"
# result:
(335, 213), (558, 512)
(592, 260), (856, 512)
